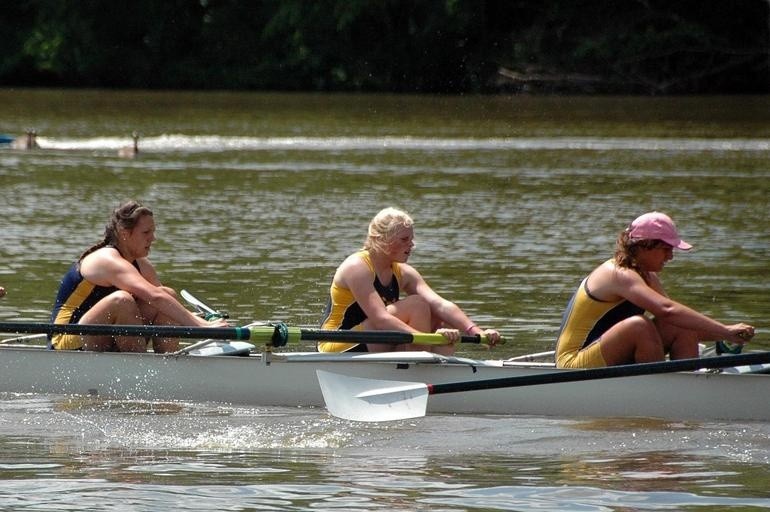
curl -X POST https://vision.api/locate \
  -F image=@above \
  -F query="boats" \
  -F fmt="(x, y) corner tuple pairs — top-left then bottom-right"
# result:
(1, 315), (770, 427)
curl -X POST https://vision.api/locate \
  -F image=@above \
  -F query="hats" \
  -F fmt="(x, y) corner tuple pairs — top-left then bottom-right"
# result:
(626, 212), (694, 252)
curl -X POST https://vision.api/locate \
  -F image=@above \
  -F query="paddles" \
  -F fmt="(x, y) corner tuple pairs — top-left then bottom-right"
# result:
(177, 287), (229, 339)
(315, 350), (770, 421)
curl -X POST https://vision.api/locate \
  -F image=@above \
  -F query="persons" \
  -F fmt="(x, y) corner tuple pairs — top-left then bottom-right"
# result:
(557, 211), (756, 370)
(314, 207), (503, 354)
(48, 201), (229, 355)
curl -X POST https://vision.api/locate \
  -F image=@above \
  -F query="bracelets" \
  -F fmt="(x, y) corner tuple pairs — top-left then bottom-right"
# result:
(463, 322), (477, 337)
